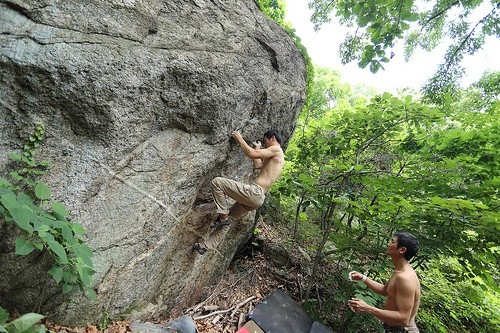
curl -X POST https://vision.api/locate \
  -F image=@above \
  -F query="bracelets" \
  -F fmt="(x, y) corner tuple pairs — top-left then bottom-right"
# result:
(362, 276), (367, 282)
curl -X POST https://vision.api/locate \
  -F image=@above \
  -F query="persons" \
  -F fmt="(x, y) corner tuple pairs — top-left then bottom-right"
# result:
(193, 128), (285, 256)
(347, 230), (420, 333)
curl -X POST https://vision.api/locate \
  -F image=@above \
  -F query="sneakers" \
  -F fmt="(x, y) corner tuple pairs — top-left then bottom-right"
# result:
(194, 241), (206, 255)
(210, 214), (233, 228)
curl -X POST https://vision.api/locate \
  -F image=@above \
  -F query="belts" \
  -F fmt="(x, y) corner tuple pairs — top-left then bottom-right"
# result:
(384, 325), (416, 331)
